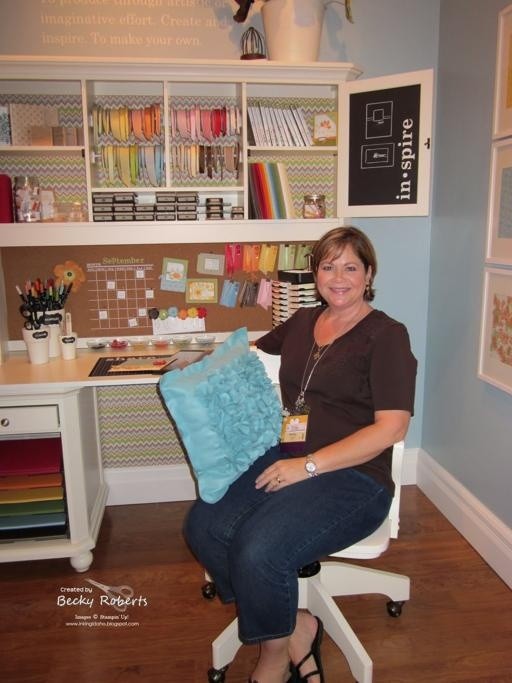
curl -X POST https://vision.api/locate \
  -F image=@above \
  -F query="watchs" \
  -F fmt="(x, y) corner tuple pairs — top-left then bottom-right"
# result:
(306, 454), (318, 478)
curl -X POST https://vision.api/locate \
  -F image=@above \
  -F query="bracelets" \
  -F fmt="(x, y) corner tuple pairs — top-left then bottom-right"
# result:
(276, 477), (281, 483)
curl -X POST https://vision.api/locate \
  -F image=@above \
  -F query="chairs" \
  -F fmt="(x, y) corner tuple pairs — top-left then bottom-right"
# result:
(175, 346), (411, 683)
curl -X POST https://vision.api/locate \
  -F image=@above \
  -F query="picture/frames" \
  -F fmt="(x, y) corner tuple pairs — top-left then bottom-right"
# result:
(336, 68), (435, 219)
(474, 3), (512, 395)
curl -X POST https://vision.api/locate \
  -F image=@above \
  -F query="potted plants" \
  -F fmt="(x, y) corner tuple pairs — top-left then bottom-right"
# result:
(232, 0), (355, 63)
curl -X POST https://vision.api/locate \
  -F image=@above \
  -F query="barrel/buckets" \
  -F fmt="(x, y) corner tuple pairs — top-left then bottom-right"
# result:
(261, 0), (323, 63)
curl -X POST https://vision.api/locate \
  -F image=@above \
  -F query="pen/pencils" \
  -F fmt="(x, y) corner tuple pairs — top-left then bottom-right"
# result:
(16, 278), (73, 311)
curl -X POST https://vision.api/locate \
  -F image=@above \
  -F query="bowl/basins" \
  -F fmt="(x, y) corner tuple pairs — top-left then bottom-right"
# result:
(108, 338), (128, 349)
(172, 334), (193, 347)
(88, 338), (107, 349)
(129, 336), (150, 349)
(196, 335), (216, 345)
(151, 337), (172, 347)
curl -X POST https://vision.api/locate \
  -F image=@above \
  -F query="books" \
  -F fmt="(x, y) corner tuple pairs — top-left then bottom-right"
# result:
(247, 101), (314, 219)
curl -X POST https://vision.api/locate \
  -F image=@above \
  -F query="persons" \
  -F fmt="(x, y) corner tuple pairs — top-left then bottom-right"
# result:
(183, 226), (418, 683)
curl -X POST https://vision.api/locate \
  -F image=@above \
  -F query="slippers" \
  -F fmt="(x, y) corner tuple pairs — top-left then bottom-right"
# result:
(248, 617), (324, 683)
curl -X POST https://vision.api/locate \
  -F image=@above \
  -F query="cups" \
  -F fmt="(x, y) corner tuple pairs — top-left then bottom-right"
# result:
(59, 335), (77, 359)
(22, 327), (49, 364)
(32, 307), (66, 358)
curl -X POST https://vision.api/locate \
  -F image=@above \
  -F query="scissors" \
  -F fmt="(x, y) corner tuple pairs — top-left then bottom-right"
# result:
(20, 298), (45, 329)
(84, 578), (134, 613)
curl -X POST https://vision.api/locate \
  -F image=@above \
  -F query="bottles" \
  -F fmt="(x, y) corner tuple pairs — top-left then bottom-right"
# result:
(13, 174), (42, 221)
(303, 193), (326, 218)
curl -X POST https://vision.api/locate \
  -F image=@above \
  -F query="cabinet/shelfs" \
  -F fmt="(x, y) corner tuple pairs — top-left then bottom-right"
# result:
(0, 53), (364, 249)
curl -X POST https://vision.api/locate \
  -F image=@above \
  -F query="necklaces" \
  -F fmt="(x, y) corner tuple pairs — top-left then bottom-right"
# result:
(295, 308), (365, 407)
(313, 316), (365, 360)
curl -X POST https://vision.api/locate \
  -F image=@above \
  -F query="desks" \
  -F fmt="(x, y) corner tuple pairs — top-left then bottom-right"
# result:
(0, 340), (283, 574)
